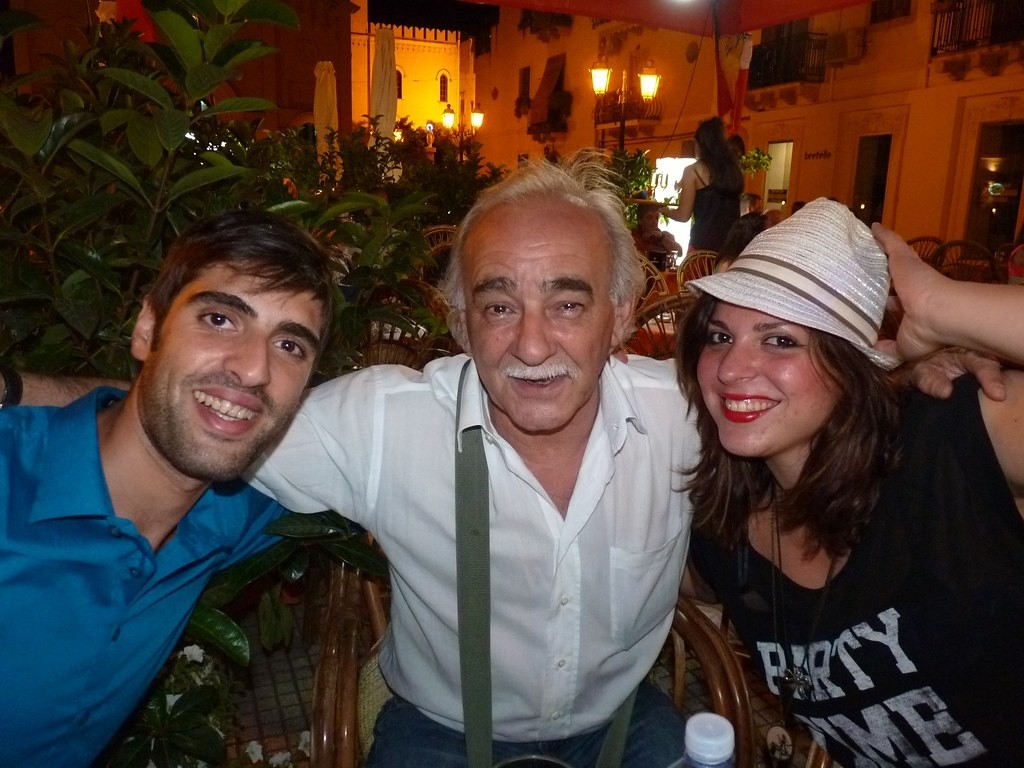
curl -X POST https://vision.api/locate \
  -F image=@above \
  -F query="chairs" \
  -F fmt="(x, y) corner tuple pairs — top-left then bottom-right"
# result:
(230, 221), (1004, 768)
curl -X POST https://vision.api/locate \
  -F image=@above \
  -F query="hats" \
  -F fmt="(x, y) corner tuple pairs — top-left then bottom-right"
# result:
(684, 197), (903, 373)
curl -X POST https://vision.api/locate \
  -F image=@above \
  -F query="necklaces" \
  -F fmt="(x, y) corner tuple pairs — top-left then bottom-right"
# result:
(769, 494), (840, 698)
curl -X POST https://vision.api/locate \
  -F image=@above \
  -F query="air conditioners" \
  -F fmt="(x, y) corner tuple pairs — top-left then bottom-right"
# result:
(830, 27), (864, 63)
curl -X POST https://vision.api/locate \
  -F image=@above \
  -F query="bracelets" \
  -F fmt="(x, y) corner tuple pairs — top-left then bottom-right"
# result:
(0, 367), (23, 408)
(660, 235), (665, 239)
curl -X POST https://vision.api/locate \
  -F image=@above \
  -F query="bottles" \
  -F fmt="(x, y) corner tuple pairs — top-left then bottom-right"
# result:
(667, 711), (735, 768)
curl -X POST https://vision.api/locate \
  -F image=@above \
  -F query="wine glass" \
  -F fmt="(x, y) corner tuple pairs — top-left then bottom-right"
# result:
(647, 184), (656, 200)
(668, 250), (678, 270)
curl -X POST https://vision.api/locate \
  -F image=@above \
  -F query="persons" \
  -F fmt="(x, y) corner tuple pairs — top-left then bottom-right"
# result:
(630, 115), (786, 273)
(0, 206), (338, 768)
(676, 196), (1024, 768)
(0, 165), (1006, 768)
